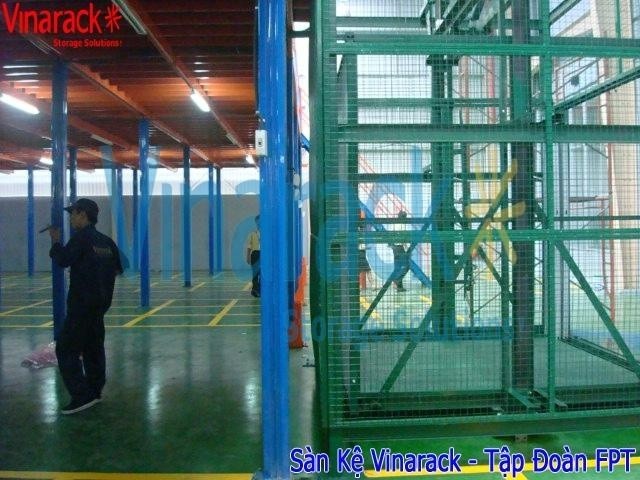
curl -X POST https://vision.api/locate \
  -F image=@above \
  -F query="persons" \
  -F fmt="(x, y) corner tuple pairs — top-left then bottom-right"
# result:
(44, 198), (130, 414)
(358, 225), (384, 291)
(244, 215), (262, 297)
(391, 211), (412, 292)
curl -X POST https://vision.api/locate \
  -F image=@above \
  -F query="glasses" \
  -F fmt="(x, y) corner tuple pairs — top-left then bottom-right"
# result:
(0, 0), (240, 150)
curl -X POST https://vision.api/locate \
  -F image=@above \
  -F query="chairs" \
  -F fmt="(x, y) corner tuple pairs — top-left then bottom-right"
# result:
(62, 391), (101, 416)
(251, 290), (260, 297)
(397, 287), (405, 292)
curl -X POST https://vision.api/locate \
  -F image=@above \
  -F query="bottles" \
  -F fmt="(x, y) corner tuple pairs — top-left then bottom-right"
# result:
(62, 199), (98, 214)
